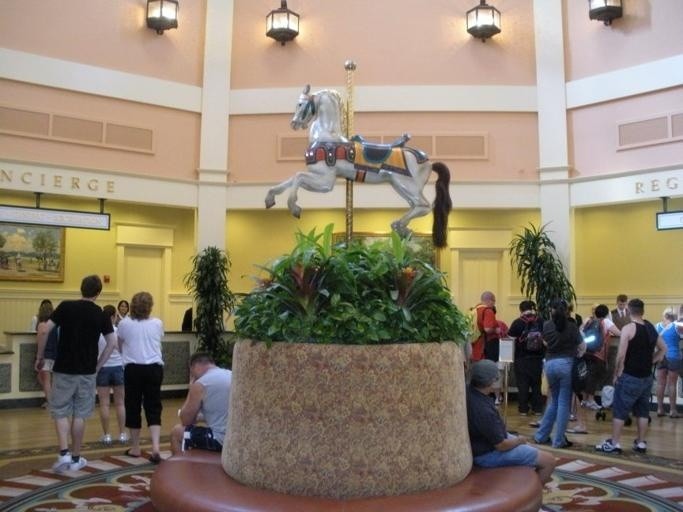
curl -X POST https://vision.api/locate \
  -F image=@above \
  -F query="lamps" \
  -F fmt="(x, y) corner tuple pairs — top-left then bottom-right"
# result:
(266, 0), (299, 46)
(465, 0), (500, 46)
(146, 0), (180, 35)
(589, 0), (623, 28)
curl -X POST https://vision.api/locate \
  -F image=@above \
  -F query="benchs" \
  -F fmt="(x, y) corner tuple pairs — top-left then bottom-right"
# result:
(152, 433), (544, 512)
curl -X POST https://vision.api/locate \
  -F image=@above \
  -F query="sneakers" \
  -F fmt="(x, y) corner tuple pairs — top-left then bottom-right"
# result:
(520, 399), (603, 447)
(632, 439), (647, 452)
(52, 452), (87, 473)
(99, 433), (160, 465)
(596, 439), (621, 454)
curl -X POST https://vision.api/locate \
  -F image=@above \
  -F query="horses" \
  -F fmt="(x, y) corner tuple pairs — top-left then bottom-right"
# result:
(265, 84), (453, 249)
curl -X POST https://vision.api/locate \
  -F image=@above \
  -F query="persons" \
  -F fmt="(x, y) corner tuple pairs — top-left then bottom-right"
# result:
(467, 358), (558, 490)
(534, 296), (588, 450)
(169, 348), (233, 457)
(32, 297), (130, 449)
(114, 290), (166, 463)
(594, 297), (669, 455)
(468, 291), (683, 424)
(32, 272), (118, 476)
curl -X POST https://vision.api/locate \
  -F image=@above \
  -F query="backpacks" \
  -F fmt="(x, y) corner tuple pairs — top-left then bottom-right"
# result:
(583, 316), (604, 352)
(519, 315), (544, 352)
(467, 304), (488, 343)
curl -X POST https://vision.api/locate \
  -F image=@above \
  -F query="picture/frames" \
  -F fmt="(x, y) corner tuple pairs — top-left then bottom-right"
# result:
(0, 221), (66, 284)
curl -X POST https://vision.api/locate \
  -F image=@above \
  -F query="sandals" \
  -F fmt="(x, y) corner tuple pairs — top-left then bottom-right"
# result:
(657, 408), (682, 417)
